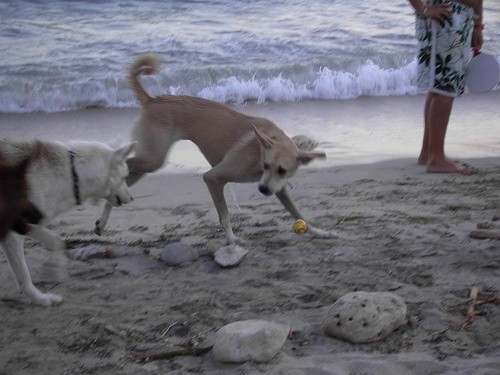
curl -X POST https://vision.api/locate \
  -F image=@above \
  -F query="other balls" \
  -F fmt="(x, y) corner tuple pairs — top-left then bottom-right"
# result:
(292, 219), (307, 234)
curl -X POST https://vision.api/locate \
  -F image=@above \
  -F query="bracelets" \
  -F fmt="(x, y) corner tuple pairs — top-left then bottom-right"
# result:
(475, 23), (484, 30)
(420, 6), (428, 16)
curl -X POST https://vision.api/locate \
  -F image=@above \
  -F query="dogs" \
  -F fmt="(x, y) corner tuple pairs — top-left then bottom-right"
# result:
(0, 138), (138, 306)
(94, 54), (340, 245)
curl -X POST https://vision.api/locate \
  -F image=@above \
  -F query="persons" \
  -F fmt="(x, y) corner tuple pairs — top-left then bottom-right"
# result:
(411, 0), (485, 174)
